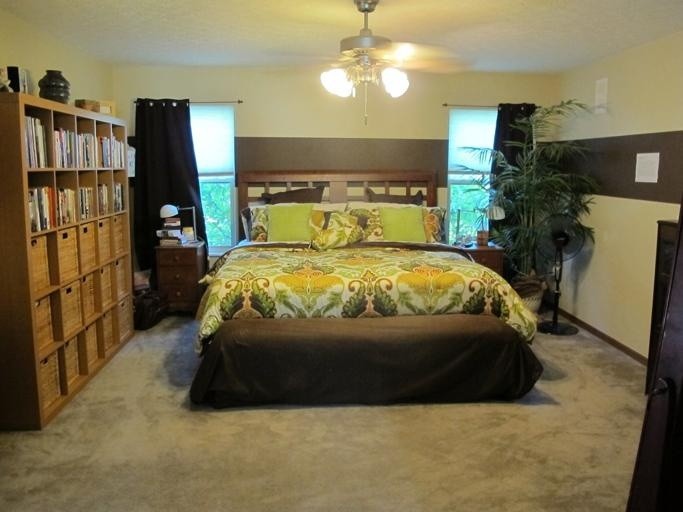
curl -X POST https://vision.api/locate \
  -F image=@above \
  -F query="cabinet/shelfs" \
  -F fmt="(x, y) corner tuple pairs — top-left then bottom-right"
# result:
(1, 88), (136, 432)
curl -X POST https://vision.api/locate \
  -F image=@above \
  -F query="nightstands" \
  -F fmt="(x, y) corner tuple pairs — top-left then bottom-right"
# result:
(154, 241), (205, 316)
(460, 239), (504, 279)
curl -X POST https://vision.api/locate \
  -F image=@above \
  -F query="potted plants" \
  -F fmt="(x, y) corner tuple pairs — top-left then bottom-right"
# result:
(454, 98), (595, 314)
(473, 206), (489, 245)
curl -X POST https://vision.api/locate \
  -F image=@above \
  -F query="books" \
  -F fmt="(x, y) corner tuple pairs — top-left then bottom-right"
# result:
(156, 216), (204, 248)
(25, 116), (125, 233)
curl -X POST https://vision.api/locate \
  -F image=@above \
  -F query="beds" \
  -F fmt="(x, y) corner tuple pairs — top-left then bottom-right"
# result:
(190, 170), (543, 410)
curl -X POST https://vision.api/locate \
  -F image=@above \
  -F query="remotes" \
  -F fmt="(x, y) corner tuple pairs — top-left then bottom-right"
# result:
(465, 243), (473, 247)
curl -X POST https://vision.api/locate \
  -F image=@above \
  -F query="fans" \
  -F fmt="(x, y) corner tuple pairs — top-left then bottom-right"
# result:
(536, 213), (585, 337)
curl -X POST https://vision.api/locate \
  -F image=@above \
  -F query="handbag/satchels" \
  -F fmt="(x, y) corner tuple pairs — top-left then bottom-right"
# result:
(135, 291), (169, 330)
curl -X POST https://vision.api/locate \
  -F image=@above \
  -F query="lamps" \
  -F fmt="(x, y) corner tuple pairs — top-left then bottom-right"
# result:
(318, 1), (411, 125)
(454, 206), (506, 246)
(159, 204), (196, 241)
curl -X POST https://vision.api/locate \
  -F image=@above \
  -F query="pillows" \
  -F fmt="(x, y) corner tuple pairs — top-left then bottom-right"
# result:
(239, 186), (446, 252)
